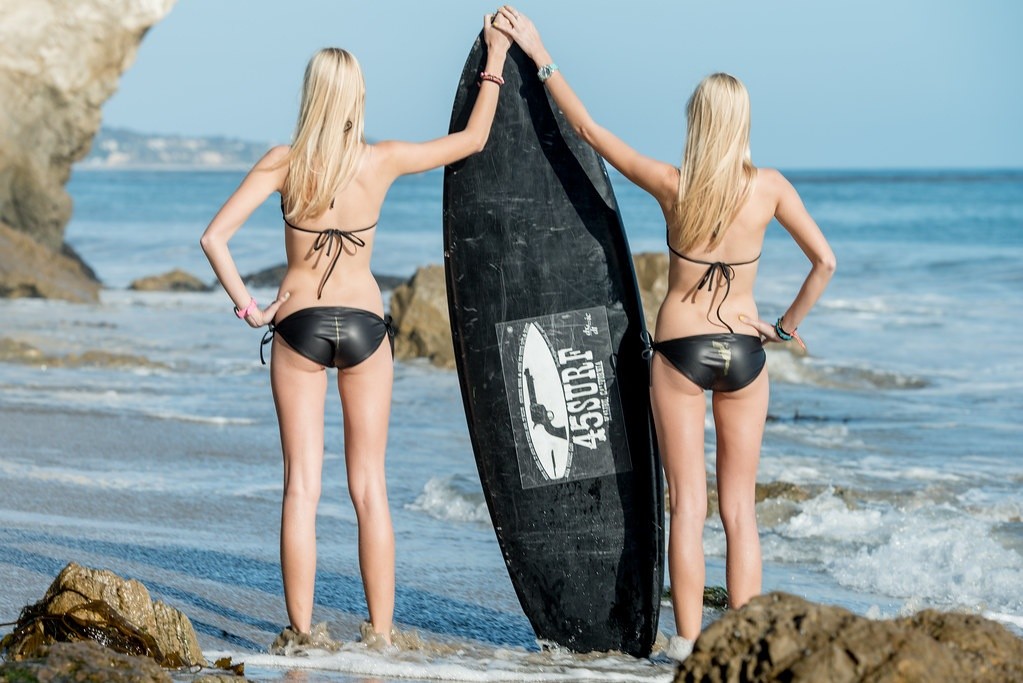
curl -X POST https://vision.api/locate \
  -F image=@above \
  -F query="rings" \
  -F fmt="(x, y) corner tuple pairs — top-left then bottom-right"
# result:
(515, 13), (520, 19)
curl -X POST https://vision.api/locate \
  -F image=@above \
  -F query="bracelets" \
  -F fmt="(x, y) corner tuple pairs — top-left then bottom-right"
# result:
(477, 71), (505, 88)
(775, 315), (807, 351)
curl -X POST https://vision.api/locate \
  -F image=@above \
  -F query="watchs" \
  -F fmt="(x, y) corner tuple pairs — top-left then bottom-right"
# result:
(536, 63), (559, 84)
(233, 297), (257, 320)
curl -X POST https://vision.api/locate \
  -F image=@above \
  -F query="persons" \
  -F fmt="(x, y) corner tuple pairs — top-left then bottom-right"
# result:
(198, 10), (514, 647)
(493, 5), (836, 643)
